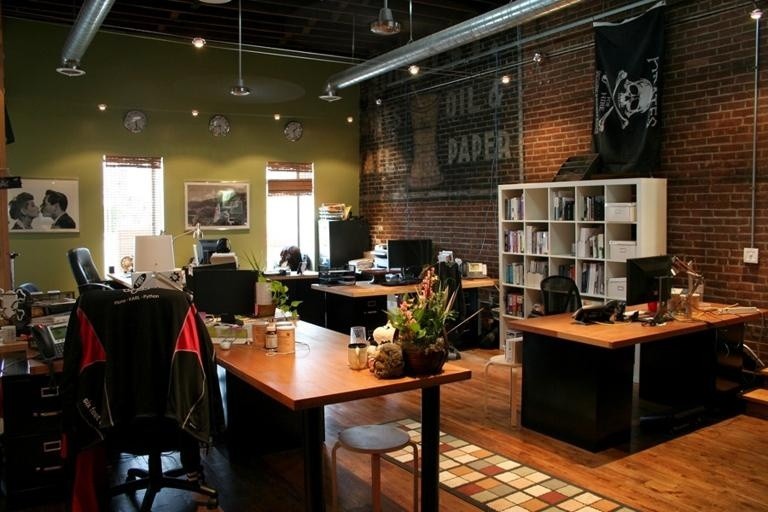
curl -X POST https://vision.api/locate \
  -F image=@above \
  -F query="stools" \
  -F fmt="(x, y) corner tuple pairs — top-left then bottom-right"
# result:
(483, 354), (523, 428)
(331, 425), (418, 512)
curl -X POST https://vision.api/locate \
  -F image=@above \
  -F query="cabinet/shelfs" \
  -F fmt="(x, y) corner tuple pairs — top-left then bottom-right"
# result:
(318, 219), (372, 282)
(498, 176), (669, 352)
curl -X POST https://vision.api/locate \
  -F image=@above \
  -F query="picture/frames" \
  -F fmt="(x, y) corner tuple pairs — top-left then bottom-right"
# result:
(7, 177), (80, 233)
(184, 181), (249, 231)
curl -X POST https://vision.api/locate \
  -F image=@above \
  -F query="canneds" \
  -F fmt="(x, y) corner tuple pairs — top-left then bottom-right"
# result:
(252, 323), (269, 346)
(275, 323), (292, 328)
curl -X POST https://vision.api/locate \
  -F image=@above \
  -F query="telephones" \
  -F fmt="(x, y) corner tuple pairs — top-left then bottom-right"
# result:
(460, 262), (487, 279)
(31, 322), (68, 362)
(572, 300), (618, 324)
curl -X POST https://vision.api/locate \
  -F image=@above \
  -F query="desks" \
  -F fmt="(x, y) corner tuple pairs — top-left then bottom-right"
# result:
(0, 341), (79, 508)
(310, 279), (499, 350)
(261, 270), (326, 327)
(506, 298), (768, 453)
(107, 272), (132, 290)
(197, 309), (472, 512)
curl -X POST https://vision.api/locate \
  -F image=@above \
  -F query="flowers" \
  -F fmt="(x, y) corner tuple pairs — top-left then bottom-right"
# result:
(380, 265), (484, 347)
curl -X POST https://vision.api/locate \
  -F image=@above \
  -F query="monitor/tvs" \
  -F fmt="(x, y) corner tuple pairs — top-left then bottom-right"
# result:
(192, 267), (259, 326)
(387, 238), (432, 284)
(199, 239), (227, 263)
(626, 255), (673, 323)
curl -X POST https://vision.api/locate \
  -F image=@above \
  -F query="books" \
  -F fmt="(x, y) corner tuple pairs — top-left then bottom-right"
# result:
(504, 190), (605, 315)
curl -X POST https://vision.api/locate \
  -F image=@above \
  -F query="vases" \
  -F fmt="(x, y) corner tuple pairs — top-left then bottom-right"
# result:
(399, 349), (449, 376)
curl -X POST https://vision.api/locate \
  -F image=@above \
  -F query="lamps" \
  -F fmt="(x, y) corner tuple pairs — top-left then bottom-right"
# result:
(668, 255), (704, 306)
(230, 1), (420, 105)
(172, 223), (204, 242)
(134, 235), (175, 275)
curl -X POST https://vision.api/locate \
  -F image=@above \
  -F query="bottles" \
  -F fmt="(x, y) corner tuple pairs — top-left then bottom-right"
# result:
(265, 327), (277, 349)
(0, 330), (8, 344)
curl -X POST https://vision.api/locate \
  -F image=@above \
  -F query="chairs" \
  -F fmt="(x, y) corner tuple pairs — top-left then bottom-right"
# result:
(540, 275), (582, 317)
(66, 246), (131, 293)
(63, 288), (225, 511)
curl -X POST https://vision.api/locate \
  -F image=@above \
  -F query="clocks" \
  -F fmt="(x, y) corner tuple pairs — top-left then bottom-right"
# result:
(209, 114), (231, 138)
(123, 110), (147, 134)
(283, 121), (304, 142)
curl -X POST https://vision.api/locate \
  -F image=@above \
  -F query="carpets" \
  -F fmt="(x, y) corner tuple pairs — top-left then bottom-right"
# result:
(377, 416), (638, 512)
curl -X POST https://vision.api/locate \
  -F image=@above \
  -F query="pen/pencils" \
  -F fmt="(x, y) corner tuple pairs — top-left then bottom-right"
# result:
(0, 359), (4, 377)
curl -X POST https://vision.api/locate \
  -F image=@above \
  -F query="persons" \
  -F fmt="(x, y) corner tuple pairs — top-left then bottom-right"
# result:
(11, 189), (75, 229)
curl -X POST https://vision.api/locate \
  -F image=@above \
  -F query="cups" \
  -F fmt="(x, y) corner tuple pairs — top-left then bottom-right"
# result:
(350, 326), (367, 344)
(275, 323), (292, 326)
(348, 343), (368, 369)
